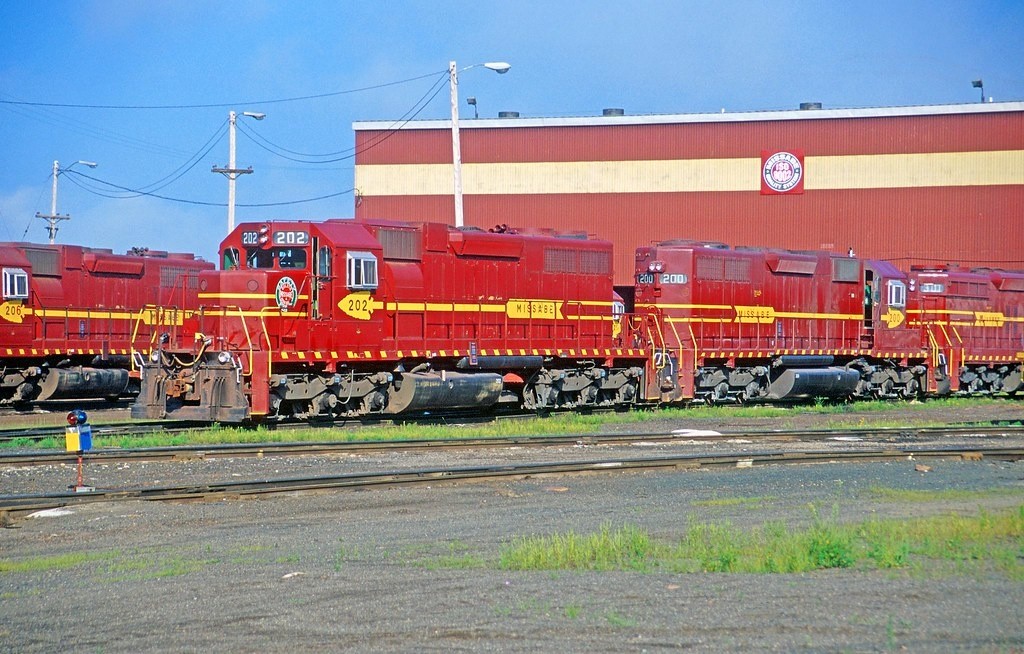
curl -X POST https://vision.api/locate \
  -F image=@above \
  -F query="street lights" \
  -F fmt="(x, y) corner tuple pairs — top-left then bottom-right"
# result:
(49, 160), (98, 246)
(449, 60), (512, 228)
(228, 111), (268, 234)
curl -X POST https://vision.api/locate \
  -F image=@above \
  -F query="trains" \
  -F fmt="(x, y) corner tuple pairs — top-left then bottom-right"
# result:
(0, 241), (216, 412)
(129, 214), (1023, 430)
